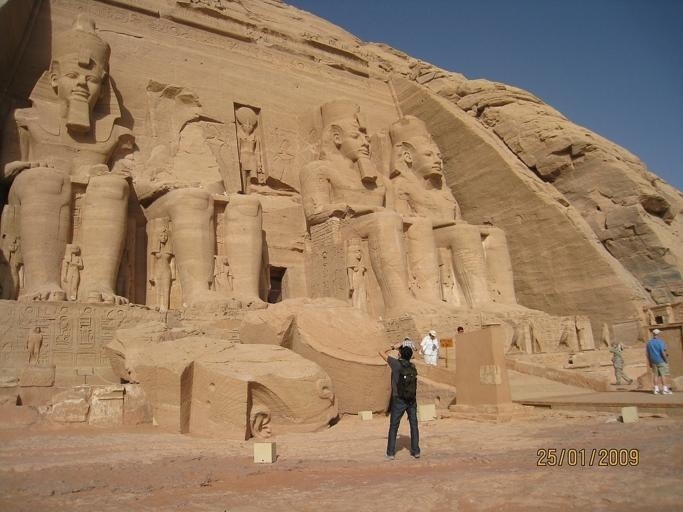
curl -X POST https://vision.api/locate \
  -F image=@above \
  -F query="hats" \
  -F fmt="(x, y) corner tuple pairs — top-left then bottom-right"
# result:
(429, 330), (437, 337)
(653, 329), (663, 334)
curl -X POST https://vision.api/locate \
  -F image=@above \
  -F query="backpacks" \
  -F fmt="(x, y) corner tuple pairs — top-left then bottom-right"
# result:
(398, 359), (417, 401)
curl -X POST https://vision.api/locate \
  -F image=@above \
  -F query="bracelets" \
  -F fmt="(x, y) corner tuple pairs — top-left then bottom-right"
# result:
(391, 346), (394, 350)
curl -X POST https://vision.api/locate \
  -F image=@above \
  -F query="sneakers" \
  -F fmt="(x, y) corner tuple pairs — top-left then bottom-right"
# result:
(654, 391), (673, 395)
(385, 448), (395, 460)
(627, 379), (633, 385)
(408, 449), (420, 458)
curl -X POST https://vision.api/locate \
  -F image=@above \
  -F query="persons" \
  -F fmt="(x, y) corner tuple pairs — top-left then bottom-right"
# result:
(419, 330), (439, 366)
(0, 29), (135, 304)
(647, 329), (673, 395)
(610, 342), (633, 385)
(403, 338), (417, 359)
(237, 123), (258, 194)
(458, 327), (464, 335)
(379, 343), (420, 459)
(300, 99), (443, 319)
(390, 115), (517, 308)
(146, 188), (262, 311)
